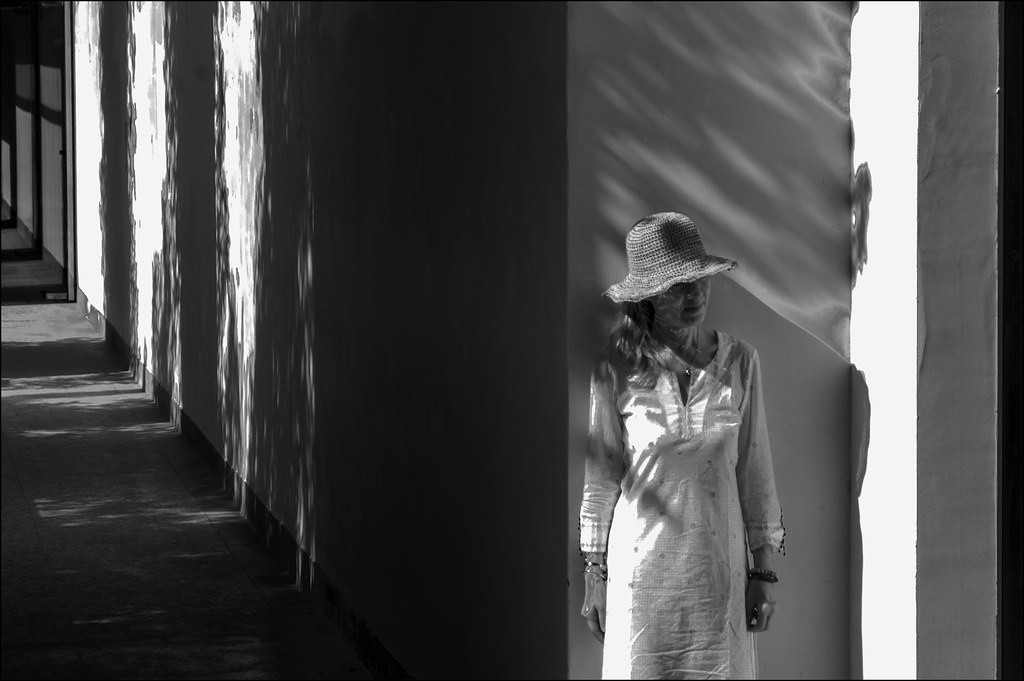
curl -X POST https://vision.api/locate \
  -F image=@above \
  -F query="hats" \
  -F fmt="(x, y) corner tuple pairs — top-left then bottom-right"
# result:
(601, 212), (738, 303)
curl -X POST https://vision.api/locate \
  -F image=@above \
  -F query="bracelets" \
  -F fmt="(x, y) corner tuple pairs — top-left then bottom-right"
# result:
(583, 558), (608, 581)
(749, 567), (779, 585)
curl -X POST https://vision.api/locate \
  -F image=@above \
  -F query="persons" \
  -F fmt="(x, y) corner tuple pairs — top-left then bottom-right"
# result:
(576, 213), (786, 681)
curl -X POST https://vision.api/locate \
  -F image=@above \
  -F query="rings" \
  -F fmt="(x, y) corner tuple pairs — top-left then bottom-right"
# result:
(585, 608), (589, 612)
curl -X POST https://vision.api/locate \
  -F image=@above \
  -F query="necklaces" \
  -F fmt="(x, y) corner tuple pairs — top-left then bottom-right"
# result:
(664, 323), (700, 375)
